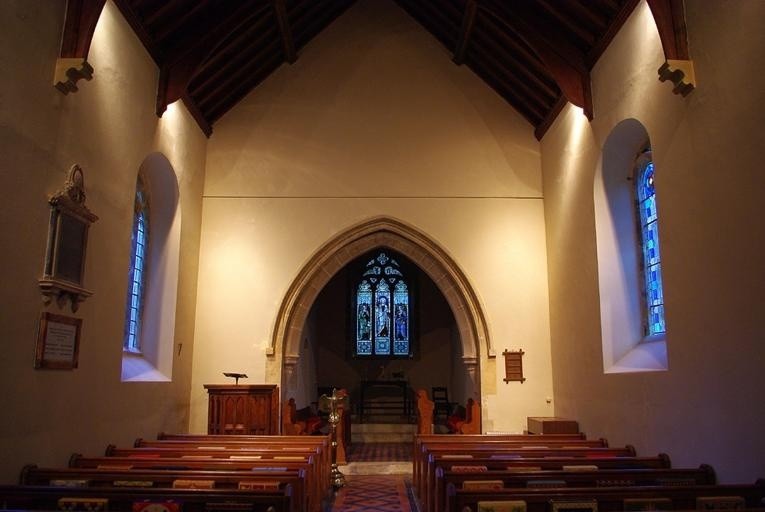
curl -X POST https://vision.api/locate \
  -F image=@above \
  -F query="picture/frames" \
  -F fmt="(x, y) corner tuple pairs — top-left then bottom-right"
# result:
(34, 312), (84, 369)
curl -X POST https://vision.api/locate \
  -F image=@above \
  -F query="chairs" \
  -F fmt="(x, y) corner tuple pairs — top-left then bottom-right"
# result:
(431, 387), (453, 419)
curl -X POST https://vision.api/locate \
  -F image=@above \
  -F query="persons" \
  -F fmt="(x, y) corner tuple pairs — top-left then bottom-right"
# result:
(358, 304), (371, 340)
(376, 297), (389, 337)
(394, 303), (407, 340)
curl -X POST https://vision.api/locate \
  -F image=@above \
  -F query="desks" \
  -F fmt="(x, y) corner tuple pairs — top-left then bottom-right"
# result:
(356, 378), (411, 417)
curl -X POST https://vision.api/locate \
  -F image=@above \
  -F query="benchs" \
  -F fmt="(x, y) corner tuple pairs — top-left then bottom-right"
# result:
(0, 433), (333, 512)
(411, 434), (765, 512)
(282, 397), (325, 435)
(447, 398), (480, 433)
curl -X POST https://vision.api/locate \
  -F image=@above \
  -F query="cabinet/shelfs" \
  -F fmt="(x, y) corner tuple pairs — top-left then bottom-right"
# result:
(526, 417), (578, 434)
